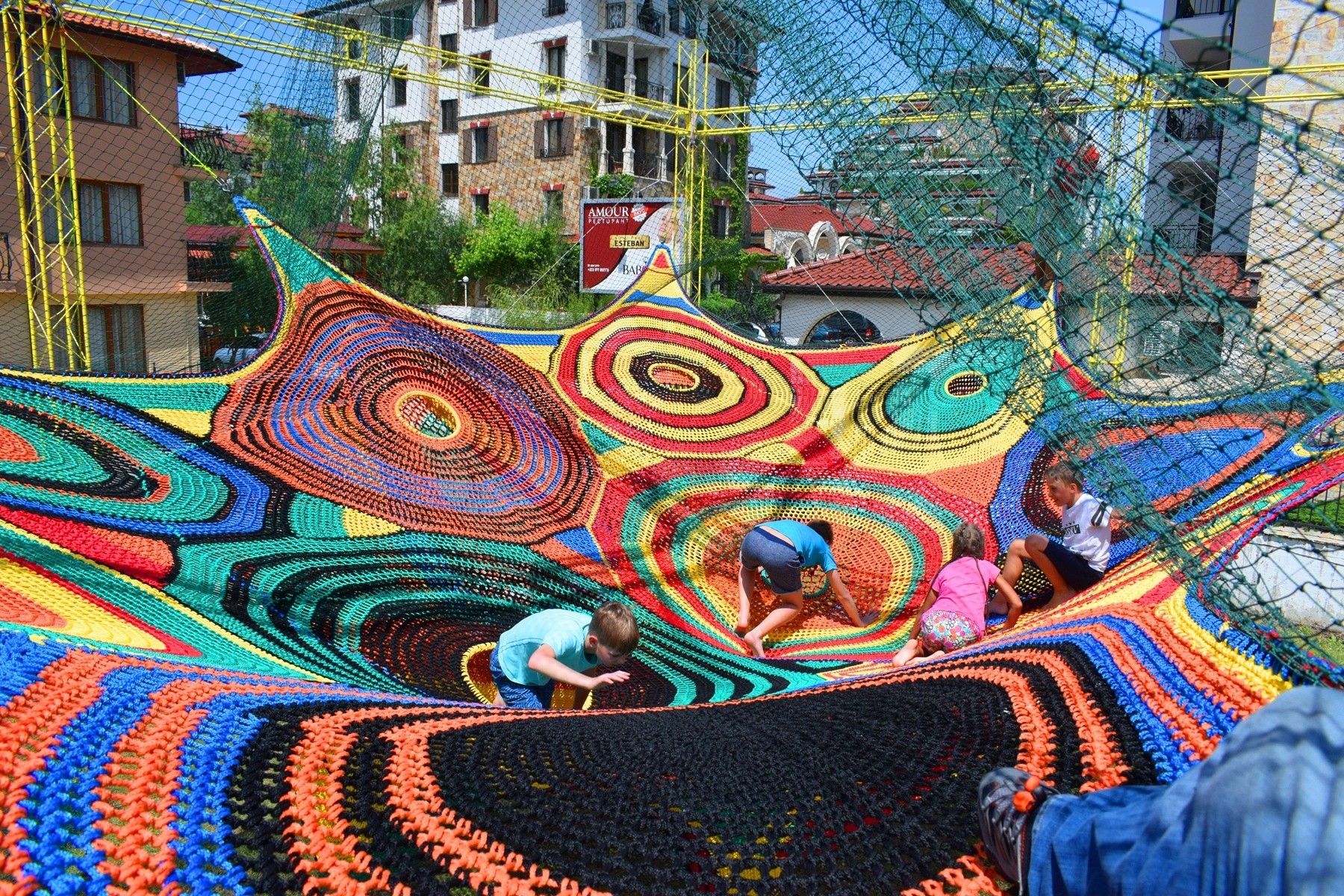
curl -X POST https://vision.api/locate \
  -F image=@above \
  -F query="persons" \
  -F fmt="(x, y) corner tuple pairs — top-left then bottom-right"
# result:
(489, 602), (640, 708)
(735, 519), (882, 658)
(892, 523), (1022, 667)
(983, 461), (1203, 614)
(980, 684), (1344, 896)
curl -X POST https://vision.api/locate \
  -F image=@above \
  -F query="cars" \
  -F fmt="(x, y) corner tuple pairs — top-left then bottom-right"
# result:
(732, 321), (769, 342)
(197, 317), (218, 337)
(762, 321), (842, 344)
(212, 333), (271, 371)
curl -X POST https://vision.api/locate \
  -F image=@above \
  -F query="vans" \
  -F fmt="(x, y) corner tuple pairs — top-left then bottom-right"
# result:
(840, 310), (883, 344)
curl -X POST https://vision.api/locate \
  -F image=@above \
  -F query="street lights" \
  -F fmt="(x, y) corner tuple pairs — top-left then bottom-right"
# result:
(461, 276), (469, 308)
